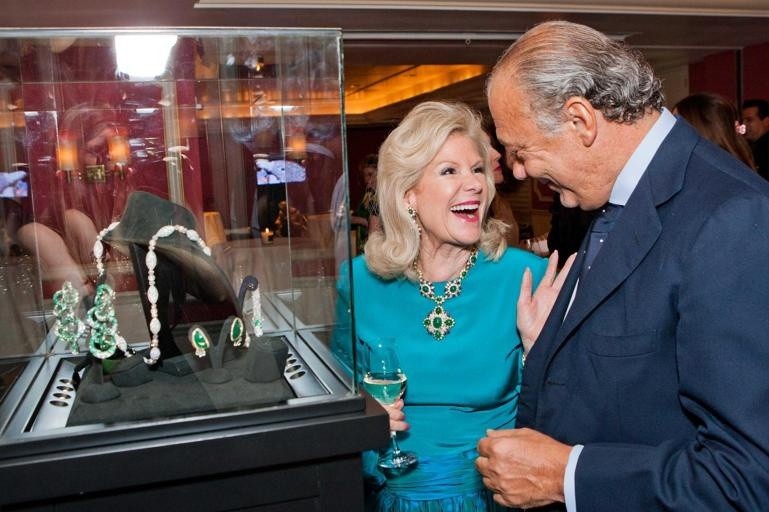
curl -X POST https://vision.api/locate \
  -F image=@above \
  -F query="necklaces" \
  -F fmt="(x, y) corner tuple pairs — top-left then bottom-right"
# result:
(409, 236), (484, 343)
(91, 211), (211, 366)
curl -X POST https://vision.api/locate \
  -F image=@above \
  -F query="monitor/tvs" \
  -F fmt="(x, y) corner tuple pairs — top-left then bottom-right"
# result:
(251, 149), (307, 186)
(0, 168), (30, 199)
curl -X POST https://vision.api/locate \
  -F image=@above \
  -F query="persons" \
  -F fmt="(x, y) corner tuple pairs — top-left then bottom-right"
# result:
(274, 199), (311, 238)
(470, 18), (768, 511)
(11, 96), (143, 349)
(330, 168), (350, 274)
(482, 130), (550, 257)
(740, 99), (768, 180)
(351, 157), (380, 257)
(326, 94), (577, 511)
(672, 93), (757, 172)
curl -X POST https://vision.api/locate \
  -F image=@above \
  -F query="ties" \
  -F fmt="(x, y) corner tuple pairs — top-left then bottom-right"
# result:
(575, 204), (623, 302)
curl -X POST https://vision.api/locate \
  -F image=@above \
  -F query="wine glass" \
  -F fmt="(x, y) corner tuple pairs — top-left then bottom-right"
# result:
(361, 338), (420, 472)
